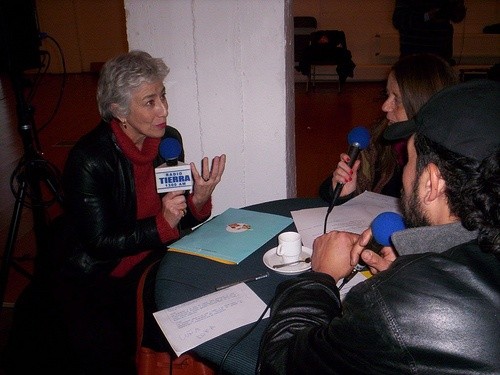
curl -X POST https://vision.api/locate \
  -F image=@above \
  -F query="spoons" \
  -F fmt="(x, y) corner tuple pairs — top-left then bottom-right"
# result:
(273, 257), (311, 268)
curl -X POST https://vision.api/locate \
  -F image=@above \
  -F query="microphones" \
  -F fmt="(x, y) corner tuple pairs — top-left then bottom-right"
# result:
(343, 212), (406, 284)
(155, 138), (194, 194)
(328, 127), (371, 213)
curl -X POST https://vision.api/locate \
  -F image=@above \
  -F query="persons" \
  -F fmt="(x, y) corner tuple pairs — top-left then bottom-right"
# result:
(62, 50), (227, 278)
(320, 0), (467, 206)
(255, 78), (500, 375)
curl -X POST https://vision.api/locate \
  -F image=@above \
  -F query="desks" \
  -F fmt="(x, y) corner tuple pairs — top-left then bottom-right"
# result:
(154, 195), (403, 375)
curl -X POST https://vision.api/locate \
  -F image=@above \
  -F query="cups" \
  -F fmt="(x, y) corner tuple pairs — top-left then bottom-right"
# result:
(277, 232), (301, 265)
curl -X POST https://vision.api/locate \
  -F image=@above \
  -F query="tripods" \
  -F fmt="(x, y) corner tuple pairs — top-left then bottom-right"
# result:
(0, 70), (64, 308)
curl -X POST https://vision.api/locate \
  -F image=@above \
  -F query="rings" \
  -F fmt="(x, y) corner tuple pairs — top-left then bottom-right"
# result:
(180, 207), (185, 216)
(202, 176), (210, 182)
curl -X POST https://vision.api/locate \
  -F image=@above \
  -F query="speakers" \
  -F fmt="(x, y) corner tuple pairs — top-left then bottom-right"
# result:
(0, 0), (42, 74)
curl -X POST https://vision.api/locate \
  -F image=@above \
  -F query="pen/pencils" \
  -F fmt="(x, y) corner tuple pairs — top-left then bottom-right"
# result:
(215, 272), (269, 291)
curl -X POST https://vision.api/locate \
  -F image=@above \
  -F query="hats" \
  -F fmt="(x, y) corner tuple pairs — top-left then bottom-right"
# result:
(377, 78), (500, 162)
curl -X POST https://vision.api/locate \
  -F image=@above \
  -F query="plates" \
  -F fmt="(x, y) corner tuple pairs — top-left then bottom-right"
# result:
(263, 246), (312, 275)
